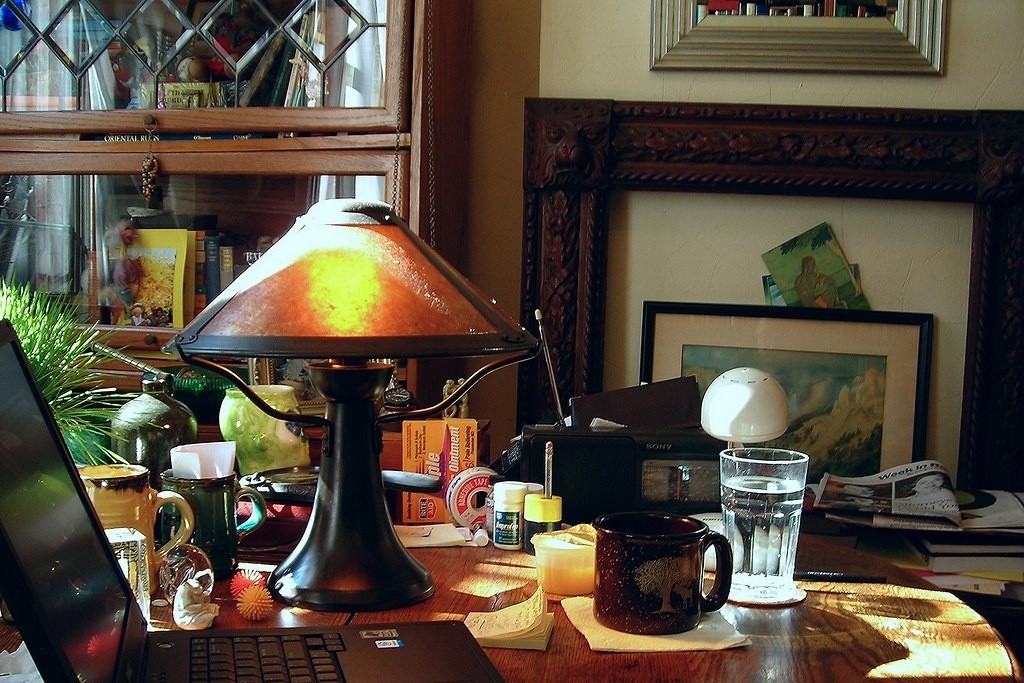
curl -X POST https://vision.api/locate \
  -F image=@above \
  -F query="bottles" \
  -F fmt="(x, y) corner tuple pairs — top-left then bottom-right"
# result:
(111, 381), (198, 543)
(494, 481), (527, 550)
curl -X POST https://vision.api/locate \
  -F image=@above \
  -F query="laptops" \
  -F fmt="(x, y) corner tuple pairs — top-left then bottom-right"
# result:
(0, 319), (509, 683)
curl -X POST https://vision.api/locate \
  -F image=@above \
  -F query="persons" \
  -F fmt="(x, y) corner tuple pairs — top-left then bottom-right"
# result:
(828, 485), (886, 517)
(131, 303), (170, 329)
(894, 471), (962, 526)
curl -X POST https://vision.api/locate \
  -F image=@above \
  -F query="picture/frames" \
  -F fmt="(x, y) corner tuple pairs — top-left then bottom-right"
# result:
(641, 301), (933, 472)
(517, 98), (1024, 493)
(650, 0), (949, 78)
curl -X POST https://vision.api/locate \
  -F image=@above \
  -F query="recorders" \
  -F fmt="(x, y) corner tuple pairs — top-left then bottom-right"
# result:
(518, 310), (724, 527)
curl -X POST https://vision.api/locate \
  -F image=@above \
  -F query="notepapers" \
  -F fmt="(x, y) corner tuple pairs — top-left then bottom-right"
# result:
(462, 583), (554, 651)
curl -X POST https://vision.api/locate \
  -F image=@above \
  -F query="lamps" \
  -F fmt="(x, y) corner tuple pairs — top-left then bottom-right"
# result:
(162, 197), (538, 612)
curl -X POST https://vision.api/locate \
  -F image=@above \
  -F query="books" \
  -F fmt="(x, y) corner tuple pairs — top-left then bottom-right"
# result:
(240, 0), (318, 137)
(0, 19), (120, 109)
(193, 229), (264, 321)
(926, 538), (1024, 583)
(464, 586), (555, 650)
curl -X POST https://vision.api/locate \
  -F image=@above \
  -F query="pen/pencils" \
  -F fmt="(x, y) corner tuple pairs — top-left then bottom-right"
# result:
(792, 570), (888, 583)
(544, 440), (554, 534)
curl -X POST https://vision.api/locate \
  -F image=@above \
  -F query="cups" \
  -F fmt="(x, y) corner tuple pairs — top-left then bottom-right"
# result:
(720, 448), (809, 602)
(160, 469), (268, 580)
(592, 512), (733, 635)
(78, 464), (195, 596)
(531, 536), (595, 595)
